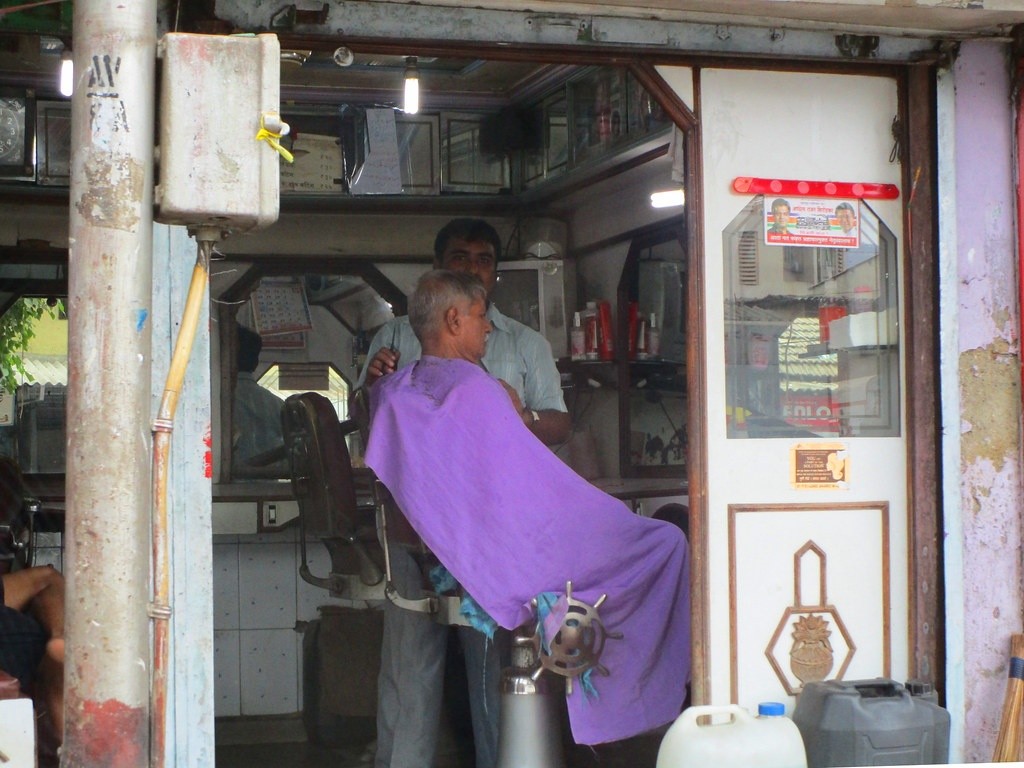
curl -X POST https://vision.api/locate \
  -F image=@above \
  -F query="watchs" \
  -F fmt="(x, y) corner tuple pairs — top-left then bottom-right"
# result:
(526, 408), (541, 432)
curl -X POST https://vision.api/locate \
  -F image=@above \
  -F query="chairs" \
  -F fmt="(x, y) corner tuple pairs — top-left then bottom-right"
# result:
(279, 391), (389, 604)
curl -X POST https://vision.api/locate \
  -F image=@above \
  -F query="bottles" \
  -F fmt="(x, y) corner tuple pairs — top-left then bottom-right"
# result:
(655, 702), (807, 768)
(583, 301), (600, 360)
(570, 318), (586, 362)
(792, 677), (954, 767)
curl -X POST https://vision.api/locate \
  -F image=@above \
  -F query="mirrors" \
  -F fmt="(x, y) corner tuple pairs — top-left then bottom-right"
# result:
(617, 222), (688, 479)
(216, 258), (406, 484)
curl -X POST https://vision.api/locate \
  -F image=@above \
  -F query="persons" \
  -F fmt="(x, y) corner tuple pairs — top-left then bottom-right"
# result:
(768, 198), (794, 235)
(835, 201), (857, 237)
(360, 262), (694, 745)
(229, 319), (286, 479)
(357, 218), (573, 766)
(0, 551), (64, 762)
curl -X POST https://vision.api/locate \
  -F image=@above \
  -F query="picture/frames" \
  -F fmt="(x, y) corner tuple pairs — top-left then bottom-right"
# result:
(35, 100), (70, 187)
(440, 111), (512, 195)
(395, 114), (441, 195)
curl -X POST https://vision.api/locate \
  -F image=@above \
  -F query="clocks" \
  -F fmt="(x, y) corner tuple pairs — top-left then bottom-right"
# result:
(0, 85), (38, 182)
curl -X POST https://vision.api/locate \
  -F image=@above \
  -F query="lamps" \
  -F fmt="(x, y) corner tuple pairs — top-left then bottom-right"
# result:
(403, 55), (420, 115)
(649, 190), (685, 208)
(59, 50), (74, 97)
